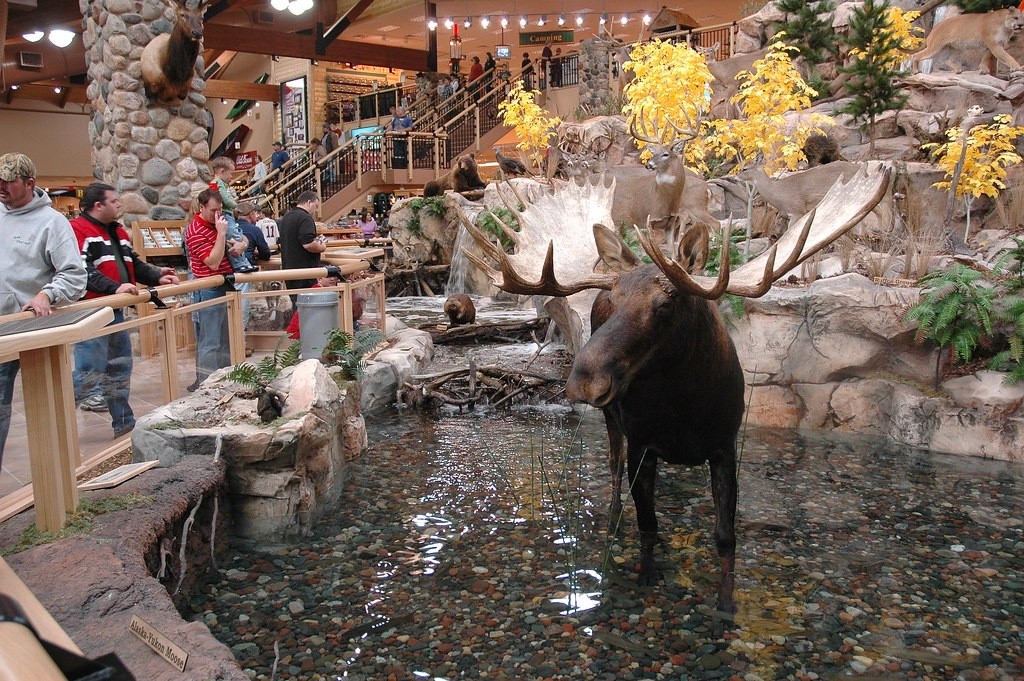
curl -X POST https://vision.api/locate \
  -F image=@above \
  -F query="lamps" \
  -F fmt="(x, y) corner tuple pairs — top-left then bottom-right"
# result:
(428, 11), (652, 31)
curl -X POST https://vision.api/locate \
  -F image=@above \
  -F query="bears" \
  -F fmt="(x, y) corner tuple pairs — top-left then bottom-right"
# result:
(423, 153), (484, 198)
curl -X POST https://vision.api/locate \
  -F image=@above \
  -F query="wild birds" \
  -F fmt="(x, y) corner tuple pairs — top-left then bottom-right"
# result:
(492, 147), (536, 179)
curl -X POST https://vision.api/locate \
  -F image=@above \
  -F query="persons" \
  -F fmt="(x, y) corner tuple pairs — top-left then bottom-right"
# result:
(68, 183), (179, 438)
(181, 157), (280, 392)
(299, 121), (345, 191)
(357, 211), (393, 259)
(541, 39), (552, 88)
(468, 56), (483, 102)
(0, 152), (87, 473)
(270, 142), (290, 188)
(484, 52), (496, 103)
(414, 72), (461, 121)
(279, 190), (362, 338)
(250, 155), (268, 196)
(550, 48), (562, 86)
(498, 63), (511, 91)
(384, 106), (412, 157)
(522, 52), (532, 91)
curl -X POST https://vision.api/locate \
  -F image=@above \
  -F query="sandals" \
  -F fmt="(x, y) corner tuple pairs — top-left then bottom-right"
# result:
(240, 265), (259, 273)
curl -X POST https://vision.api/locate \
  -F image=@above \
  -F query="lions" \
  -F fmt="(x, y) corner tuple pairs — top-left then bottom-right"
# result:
(892, 3), (1024, 77)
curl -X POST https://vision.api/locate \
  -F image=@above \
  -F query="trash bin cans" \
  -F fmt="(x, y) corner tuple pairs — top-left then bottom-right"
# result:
(373, 192), (387, 216)
(293, 293), (340, 361)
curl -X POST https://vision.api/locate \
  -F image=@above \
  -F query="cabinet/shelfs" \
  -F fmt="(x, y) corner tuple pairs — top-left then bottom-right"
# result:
(131, 220), (197, 359)
(328, 81), (390, 103)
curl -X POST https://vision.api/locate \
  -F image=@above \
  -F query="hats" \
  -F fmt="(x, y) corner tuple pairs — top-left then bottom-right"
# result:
(0, 152), (36, 180)
(236, 202), (255, 216)
(323, 124), (332, 130)
(272, 142), (281, 146)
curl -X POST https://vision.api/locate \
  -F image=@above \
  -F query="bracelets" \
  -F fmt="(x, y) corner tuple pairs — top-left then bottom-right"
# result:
(243, 241), (248, 249)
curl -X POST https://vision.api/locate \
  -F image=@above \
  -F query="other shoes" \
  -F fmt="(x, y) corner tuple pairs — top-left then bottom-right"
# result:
(113, 423), (134, 437)
(187, 380), (199, 391)
(245, 345), (254, 356)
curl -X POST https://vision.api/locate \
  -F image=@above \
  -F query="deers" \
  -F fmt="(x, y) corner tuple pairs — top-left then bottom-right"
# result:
(557, 122), (721, 247)
(454, 158), (893, 607)
(738, 151), (904, 235)
(624, 99), (706, 213)
(141, 1), (214, 110)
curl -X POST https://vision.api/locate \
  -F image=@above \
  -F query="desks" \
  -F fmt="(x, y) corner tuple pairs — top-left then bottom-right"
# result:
(317, 229), (362, 239)
(271, 248), (385, 259)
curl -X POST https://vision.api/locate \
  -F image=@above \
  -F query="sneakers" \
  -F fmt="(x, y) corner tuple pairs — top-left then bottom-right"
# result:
(81, 394), (109, 411)
